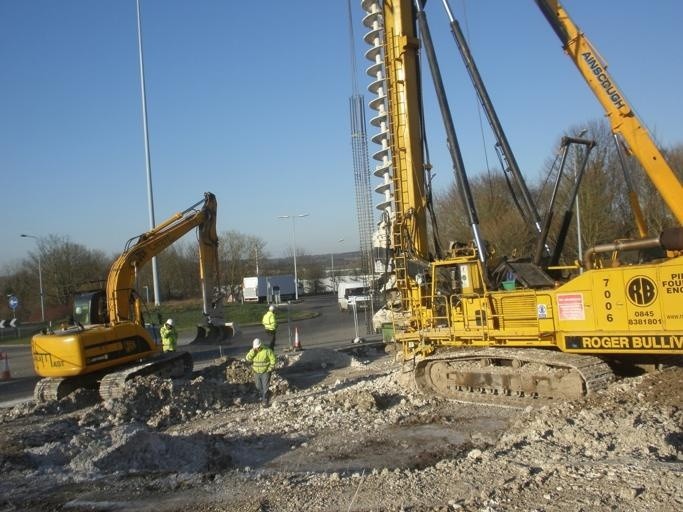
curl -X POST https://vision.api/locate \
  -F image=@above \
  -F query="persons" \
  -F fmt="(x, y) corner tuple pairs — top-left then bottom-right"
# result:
(245, 338), (276, 408)
(262, 305), (279, 350)
(75, 306), (88, 324)
(159, 318), (178, 352)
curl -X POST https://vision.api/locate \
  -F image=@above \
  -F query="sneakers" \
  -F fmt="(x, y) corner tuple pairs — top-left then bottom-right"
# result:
(260, 399), (268, 408)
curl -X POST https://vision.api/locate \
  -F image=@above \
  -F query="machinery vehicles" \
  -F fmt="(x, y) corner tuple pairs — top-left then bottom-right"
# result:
(31, 191), (242, 401)
(379, 0), (683, 412)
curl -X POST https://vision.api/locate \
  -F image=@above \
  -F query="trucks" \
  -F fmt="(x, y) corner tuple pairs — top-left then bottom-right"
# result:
(337, 281), (372, 312)
(245, 274), (305, 302)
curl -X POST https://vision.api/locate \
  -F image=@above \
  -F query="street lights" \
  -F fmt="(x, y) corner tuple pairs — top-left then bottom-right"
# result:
(330, 238), (344, 294)
(21, 235), (45, 322)
(279, 214), (307, 299)
(571, 128), (591, 276)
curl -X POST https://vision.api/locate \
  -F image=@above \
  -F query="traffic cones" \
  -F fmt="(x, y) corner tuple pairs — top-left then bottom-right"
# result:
(2, 353), (11, 381)
(293, 327), (302, 351)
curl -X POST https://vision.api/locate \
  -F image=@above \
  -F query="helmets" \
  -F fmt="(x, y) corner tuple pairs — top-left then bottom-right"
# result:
(253, 339), (262, 349)
(166, 319), (176, 327)
(269, 305), (276, 311)
